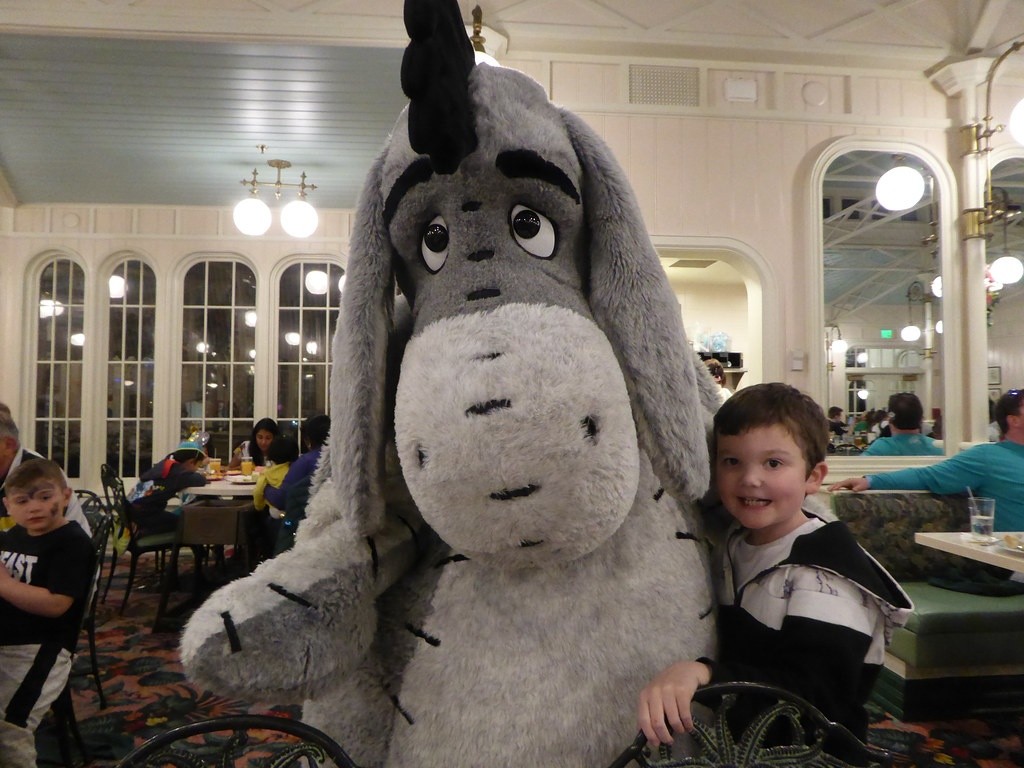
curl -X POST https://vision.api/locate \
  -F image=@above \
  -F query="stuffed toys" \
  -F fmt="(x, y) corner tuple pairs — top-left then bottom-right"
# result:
(178, 0), (750, 768)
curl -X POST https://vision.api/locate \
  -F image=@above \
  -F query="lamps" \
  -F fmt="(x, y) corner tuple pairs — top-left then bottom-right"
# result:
(984, 186), (1023, 285)
(233, 159), (318, 238)
(876, 154), (924, 210)
(900, 280), (925, 342)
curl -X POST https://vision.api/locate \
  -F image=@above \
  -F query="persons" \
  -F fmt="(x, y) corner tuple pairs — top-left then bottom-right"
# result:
(241, 414), (335, 584)
(252, 436), (300, 519)
(1, 457), (97, 768)
(227, 417), (283, 470)
(827, 389), (1024, 583)
(622, 379), (917, 768)
(0, 403), (93, 575)
(821, 391), (949, 457)
(120, 433), (214, 565)
(701, 356), (735, 403)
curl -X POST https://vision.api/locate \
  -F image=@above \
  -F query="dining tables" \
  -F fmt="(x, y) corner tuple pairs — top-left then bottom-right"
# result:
(182, 470), (259, 586)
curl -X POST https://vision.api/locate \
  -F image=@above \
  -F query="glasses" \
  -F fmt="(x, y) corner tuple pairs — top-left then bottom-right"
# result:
(1008, 388), (1021, 397)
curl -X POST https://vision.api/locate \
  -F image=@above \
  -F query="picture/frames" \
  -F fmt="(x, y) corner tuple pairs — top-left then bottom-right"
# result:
(989, 388), (1000, 403)
(988, 366), (1001, 385)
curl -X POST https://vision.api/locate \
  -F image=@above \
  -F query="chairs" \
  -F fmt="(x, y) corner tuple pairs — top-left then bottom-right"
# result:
(116, 714), (358, 768)
(73, 463), (255, 704)
(609, 681), (892, 768)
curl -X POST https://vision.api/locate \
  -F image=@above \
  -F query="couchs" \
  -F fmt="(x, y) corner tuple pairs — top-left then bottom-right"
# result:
(808, 485), (1024, 725)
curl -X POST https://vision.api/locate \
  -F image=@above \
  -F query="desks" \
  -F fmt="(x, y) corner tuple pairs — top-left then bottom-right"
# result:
(915, 532), (1024, 573)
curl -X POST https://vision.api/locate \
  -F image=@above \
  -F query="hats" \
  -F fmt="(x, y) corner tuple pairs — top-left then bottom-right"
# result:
(175, 442), (207, 457)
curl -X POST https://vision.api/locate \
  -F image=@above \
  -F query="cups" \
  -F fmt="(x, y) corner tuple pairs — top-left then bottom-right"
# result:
(968, 497), (995, 543)
(210, 459), (221, 477)
(834, 435), (840, 444)
(855, 437), (862, 446)
(241, 457), (253, 476)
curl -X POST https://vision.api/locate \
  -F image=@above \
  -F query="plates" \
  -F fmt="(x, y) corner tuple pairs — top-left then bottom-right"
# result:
(226, 476), (257, 483)
(994, 539), (1024, 553)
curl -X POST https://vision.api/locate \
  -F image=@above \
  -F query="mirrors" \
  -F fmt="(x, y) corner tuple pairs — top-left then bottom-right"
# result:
(981, 143), (1024, 442)
(804, 135), (960, 483)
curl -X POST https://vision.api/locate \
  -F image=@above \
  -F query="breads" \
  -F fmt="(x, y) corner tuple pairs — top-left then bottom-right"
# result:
(1004, 534), (1021, 548)
